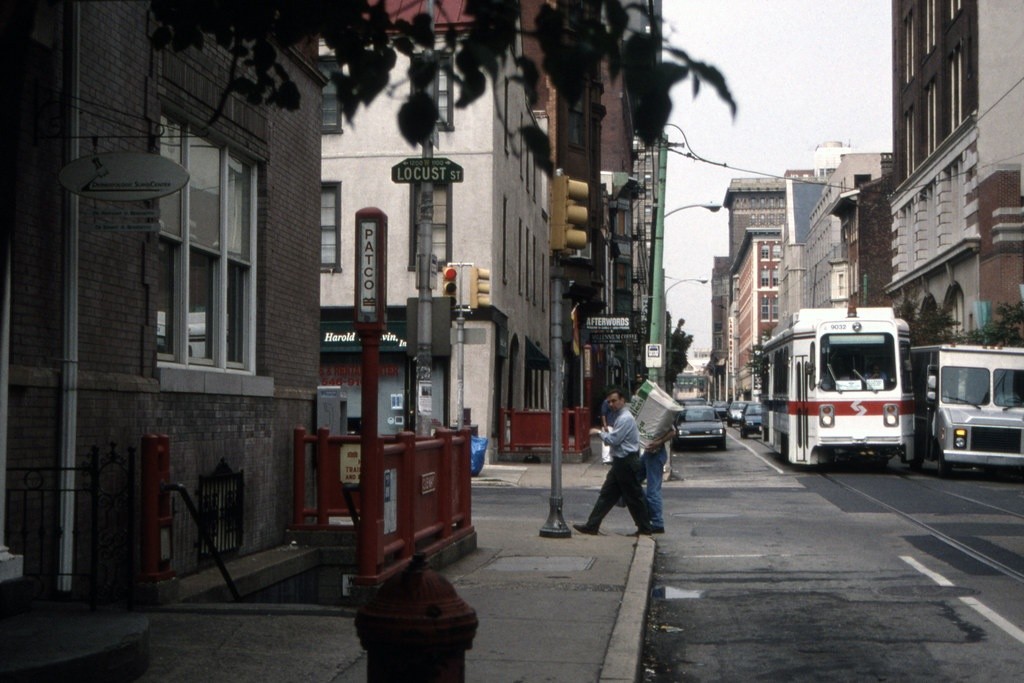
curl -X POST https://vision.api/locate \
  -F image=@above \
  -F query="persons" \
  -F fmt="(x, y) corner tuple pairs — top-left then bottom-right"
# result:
(573, 388), (654, 536)
(600, 389), (624, 464)
(618, 383), (677, 533)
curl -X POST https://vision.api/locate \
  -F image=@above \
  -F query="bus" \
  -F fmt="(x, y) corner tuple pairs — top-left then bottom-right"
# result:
(756, 305), (918, 472)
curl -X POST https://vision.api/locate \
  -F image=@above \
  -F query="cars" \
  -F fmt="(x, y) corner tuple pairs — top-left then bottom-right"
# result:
(738, 403), (762, 439)
(676, 396), (730, 420)
(726, 400), (749, 428)
(670, 404), (728, 452)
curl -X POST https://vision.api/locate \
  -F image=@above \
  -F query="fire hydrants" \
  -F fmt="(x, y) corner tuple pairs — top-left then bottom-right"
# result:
(353, 548), (480, 683)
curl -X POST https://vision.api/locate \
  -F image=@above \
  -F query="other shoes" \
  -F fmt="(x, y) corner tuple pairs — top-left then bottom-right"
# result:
(652, 527), (664, 533)
(573, 524), (598, 535)
(626, 530), (651, 537)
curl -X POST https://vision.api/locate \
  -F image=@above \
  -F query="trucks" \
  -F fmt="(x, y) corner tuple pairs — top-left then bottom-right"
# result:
(910, 344), (1024, 480)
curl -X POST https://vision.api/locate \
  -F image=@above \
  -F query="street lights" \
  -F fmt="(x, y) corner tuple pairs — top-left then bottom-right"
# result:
(658, 279), (709, 394)
(648, 201), (724, 386)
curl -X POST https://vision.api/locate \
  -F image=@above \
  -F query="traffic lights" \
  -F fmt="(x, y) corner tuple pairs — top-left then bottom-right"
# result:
(544, 175), (590, 254)
(468, 268), (490, 311)
(441, 267), (458, 309)
